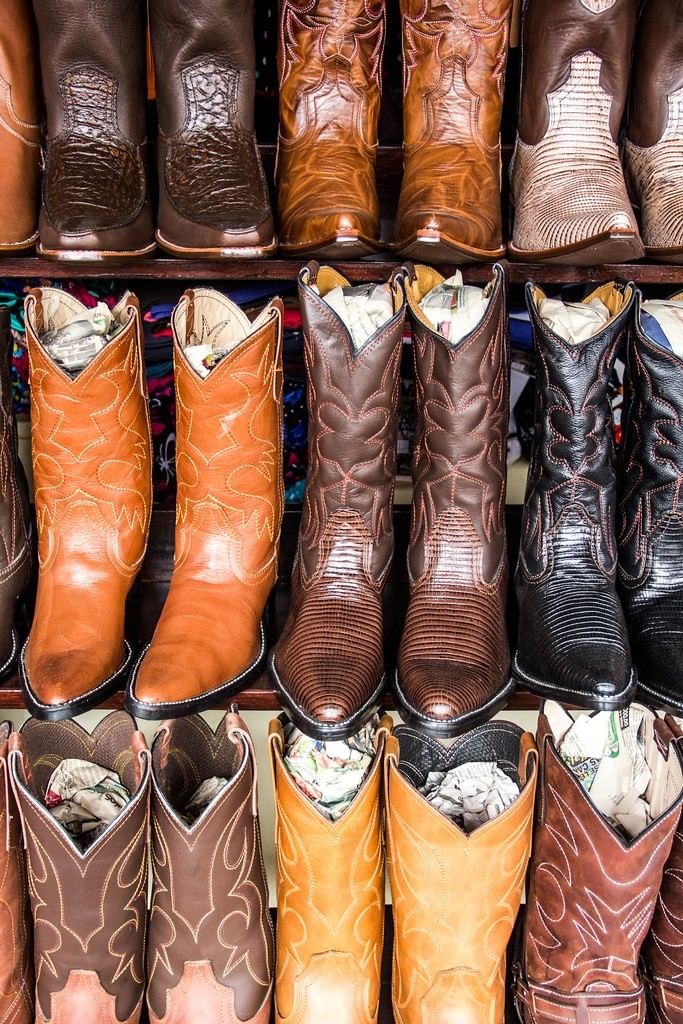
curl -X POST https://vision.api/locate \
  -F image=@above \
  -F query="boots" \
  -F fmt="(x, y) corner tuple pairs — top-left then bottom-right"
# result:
(0, 0), (683, 1024)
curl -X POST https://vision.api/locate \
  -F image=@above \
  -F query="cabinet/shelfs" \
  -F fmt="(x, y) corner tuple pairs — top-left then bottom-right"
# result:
(0, 133), (683, 712)
